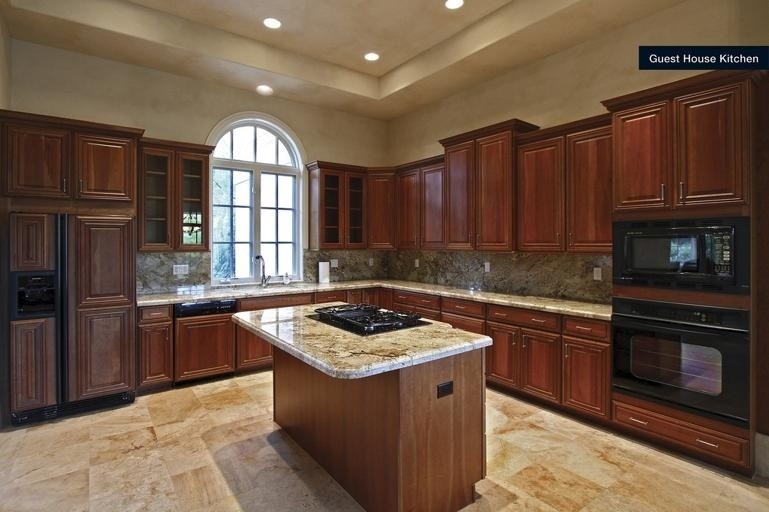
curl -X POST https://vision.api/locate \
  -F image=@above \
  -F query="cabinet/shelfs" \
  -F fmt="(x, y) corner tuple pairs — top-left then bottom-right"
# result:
(487, 303), (612, 424)
(7, 316), (58, 414)
(0, 108), (145, 213)
(68, 214), (137, 402)
(514, 114), (615, 251)
(238, 287), (485, 376)
(174, 312), (236, 385)
(137, 304), (173, 391)
(138, 134), (216, 251)
(305, 155), (447, 250)
(8, 210), (55, 271)
(598, 71), (768, 481)
(438, 113), (542, 253)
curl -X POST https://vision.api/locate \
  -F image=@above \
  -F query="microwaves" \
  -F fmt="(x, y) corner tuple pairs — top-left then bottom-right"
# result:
(10, 270), (58, 321)
(608, 219), (750, 293)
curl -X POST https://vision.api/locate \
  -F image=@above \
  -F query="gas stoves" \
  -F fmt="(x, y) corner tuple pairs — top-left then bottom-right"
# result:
(314, 303), (424, 333)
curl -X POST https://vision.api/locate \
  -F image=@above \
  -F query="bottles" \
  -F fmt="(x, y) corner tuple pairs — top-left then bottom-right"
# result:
(284, 272), (289, 285)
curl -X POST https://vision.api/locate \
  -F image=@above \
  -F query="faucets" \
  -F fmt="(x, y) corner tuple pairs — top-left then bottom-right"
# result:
(255, 255), (271, 287)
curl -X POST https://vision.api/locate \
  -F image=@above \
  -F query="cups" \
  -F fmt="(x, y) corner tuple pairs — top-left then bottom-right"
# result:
(468, 280), (475, 291)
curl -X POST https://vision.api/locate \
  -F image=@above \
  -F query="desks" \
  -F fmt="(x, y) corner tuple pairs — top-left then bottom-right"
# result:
(230, 298), (494, 511)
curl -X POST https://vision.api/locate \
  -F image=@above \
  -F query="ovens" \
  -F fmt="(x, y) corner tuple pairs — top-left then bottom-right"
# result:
(607, 302), (748, 423)
